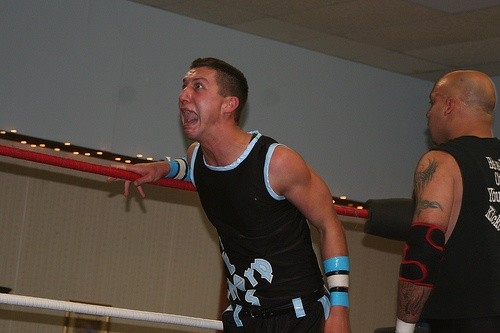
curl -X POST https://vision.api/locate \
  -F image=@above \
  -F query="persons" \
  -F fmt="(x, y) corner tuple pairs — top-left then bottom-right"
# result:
(107, 56), (351, 332)
(394, 69), (500, 333)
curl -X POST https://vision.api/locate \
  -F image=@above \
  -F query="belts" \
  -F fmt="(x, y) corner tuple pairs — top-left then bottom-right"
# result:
(232, 288), (325, 318)
